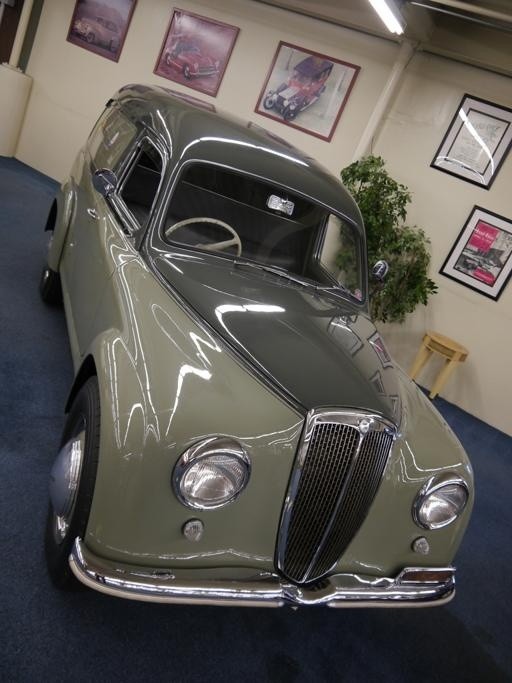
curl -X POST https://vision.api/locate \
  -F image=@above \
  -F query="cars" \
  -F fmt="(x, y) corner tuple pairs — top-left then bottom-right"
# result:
(165, 36), (220, 78)
(73, 15), (122, 52)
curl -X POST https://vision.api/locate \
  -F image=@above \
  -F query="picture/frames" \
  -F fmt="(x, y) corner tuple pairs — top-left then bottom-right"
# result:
(152, 4), (241, 96)
(437, 203), (512, 302)
(252, 39), (363, 143)
(66, 0), (139, 64)
(428, 91), (512, 191)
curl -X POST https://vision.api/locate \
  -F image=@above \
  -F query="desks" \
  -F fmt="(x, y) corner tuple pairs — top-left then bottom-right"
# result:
(406, 329), (468, 401)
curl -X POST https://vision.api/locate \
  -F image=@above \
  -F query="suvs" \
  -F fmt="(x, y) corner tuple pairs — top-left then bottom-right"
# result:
(261, 56), (334, 120)
(35, 81), (478, 611)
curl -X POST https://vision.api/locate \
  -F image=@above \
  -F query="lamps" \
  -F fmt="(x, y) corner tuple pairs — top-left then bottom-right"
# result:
(368, 1), (409, 35)
